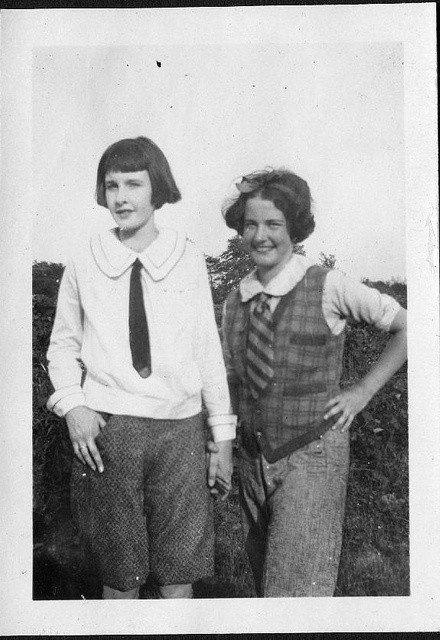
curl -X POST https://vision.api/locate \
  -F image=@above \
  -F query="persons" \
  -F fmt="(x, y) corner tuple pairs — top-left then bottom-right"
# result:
(204, 166), (407, 596)
(46, 137), (237, 599)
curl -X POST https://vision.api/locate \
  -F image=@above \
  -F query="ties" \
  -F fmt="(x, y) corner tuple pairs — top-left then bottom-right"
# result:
(129, 258), (151, 378)
(245, 292), (274, 400)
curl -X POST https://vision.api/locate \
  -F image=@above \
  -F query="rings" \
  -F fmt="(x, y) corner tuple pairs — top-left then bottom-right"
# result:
(80, 446), (88, 449)
